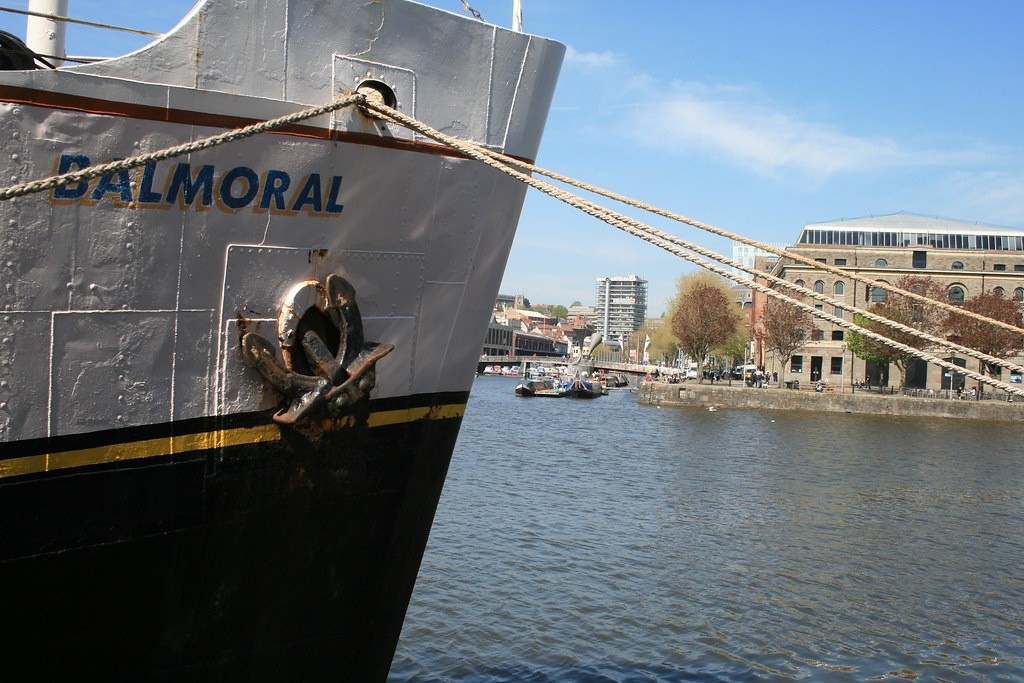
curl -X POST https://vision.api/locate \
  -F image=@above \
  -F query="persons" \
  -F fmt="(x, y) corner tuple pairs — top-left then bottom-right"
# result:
(654, 367), (778, 388)
(856, 373), (978, 399)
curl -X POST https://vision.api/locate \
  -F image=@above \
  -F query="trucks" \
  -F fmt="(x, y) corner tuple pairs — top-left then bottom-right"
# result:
(735, 364), (756, 380)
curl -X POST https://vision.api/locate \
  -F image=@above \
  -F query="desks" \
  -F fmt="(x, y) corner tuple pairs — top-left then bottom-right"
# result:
(962, 391), (971, 400)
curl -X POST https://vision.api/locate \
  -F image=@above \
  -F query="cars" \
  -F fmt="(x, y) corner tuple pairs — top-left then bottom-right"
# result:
(483, 366), (569, 376)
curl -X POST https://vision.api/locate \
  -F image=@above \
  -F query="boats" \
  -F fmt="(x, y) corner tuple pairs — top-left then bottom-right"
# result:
(515, 375), (628, 399)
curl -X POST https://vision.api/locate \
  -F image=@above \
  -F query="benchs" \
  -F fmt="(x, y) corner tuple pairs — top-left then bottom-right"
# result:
(796, 381), (833, 392)
